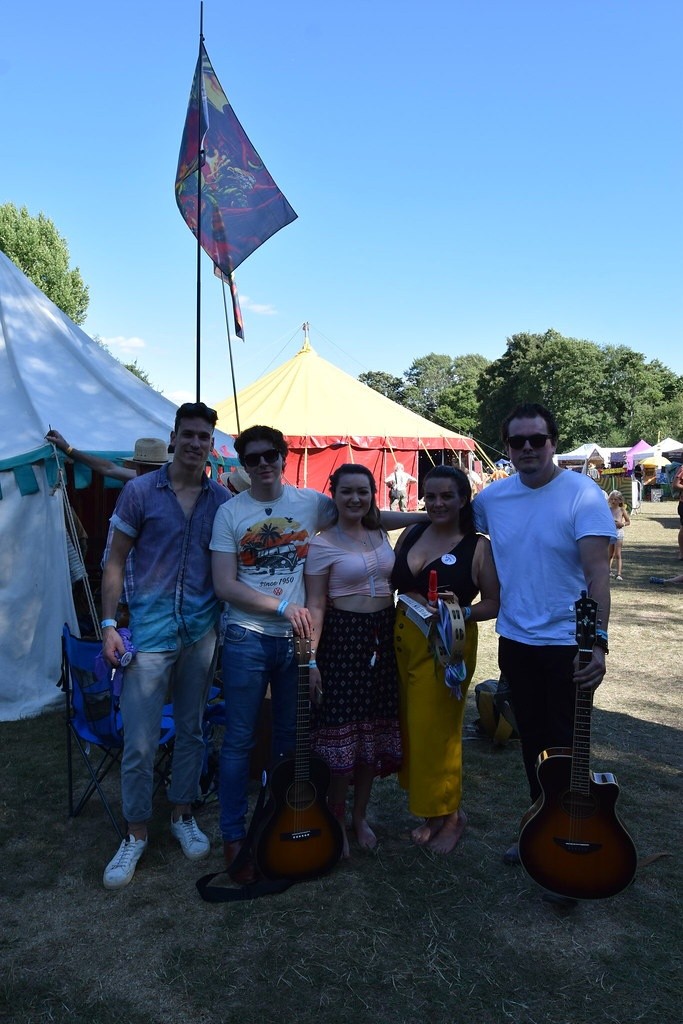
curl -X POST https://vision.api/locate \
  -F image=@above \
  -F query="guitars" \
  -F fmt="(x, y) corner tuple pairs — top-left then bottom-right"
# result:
(249, 628), (345, 881)
(515, 589), (638, 900)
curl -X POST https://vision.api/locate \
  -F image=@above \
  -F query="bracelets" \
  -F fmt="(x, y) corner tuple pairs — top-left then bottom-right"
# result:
(463, 607), (471, 621)
(596, 628), (610, 649)
(66, 446), (73, 454)
(275, 600), (288, 616)
(100, 619), (117, 629)
(308, 660), (318, 668)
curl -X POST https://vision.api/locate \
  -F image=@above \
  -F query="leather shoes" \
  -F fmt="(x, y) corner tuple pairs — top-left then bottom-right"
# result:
(223, 842), (256, 886)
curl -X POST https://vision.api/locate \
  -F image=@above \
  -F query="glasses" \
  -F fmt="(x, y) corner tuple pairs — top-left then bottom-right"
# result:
(246, 449), (281, 466)
(510, 434), (552, 449)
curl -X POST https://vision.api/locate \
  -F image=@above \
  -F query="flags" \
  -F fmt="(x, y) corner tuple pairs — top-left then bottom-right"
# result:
(174, 40), (299, 276)
(212, 204), (246, 342)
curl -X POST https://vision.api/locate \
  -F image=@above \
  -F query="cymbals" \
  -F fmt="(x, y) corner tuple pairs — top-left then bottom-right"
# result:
(433, 598), (467, 668)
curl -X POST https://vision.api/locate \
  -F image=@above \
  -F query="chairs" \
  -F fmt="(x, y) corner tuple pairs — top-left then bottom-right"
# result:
(55, 622), (228, 844)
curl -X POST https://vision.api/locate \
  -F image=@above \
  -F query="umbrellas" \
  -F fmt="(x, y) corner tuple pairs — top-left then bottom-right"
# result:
(640, 456), (672, 467)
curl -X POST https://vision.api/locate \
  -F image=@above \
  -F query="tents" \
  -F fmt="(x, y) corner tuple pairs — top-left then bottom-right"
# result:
(557, 443), (609, 475)
(632, 437), (683, 471)
(625, 439), (651, 471)
(209, 321), (476, 511)
(0, 245), (244, 723)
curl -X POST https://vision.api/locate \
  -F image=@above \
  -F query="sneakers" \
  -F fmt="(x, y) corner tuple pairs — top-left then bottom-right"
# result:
(104, 833), (148, 890)
(170, 811), (210, 861)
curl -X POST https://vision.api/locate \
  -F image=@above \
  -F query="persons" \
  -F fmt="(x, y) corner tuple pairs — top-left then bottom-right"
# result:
(673, 452), (683, 560)
(466, 402), (619, 870)
(650, 573), (683, 585)
(204, 425), (430, 871)
(489, 463), (511, 480)
(391, 464), (502, 855)
(587, 463), (601, 481)
(100, 401), (234, 890)
(383, 463), (418, 513)
(607, 490), (631, 580)
(44, 427), (252, 629)
(298, 468), (405, 862)
(464, 467), (483, 500)
(633, 460), (643, 483)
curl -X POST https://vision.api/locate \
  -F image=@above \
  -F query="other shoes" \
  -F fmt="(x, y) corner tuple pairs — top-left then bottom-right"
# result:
(506, 843), (520, 862)
(649, 577), (665, 584)
(617, 576), (623, 580)
(609, 572), (616, 576)
